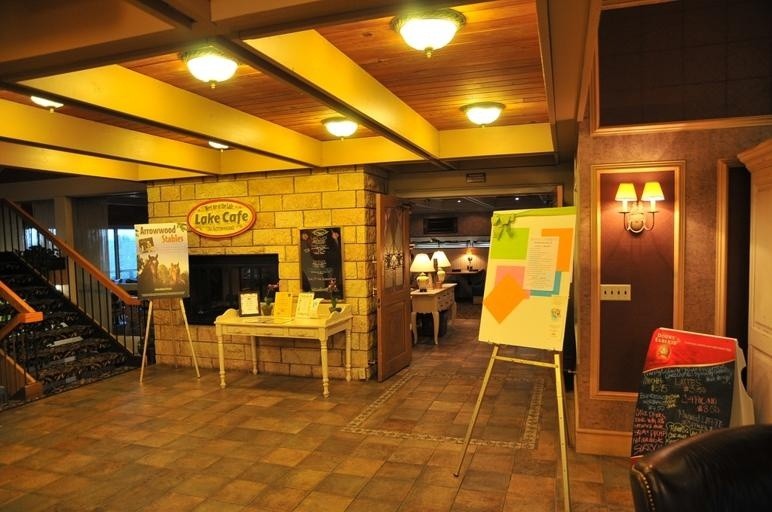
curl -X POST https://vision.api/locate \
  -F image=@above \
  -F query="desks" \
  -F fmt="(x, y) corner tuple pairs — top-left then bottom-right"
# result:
(213, 304), (354, 398)
(410, 284), (457, 345)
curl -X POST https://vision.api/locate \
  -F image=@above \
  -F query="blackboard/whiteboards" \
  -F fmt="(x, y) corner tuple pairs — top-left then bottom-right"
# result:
(298, 226), (346, 303)
(631, 326), (738, 458)
(477, 206), (577, 351)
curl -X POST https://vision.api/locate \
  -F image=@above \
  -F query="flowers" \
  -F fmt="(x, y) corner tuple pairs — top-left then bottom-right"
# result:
(266, 283), (276, 300)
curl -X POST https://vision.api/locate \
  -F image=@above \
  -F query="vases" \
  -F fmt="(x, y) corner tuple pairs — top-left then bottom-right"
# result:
(237, 291), (261, 318)
(260, 304), (274, 316)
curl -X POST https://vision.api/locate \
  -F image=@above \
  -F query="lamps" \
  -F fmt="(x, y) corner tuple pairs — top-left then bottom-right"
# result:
(411, 253), (437, 292)
(390, 10), (466, 59)
(431, 250), (452, 282)
(322, 118), (359, 143)
(615, 181), (664, 233)
(180, 45), (239, 91)
(462, 100), (506, 130)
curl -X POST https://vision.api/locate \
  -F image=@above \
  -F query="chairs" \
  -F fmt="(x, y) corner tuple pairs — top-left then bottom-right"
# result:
(632, 424), (772, 512)
